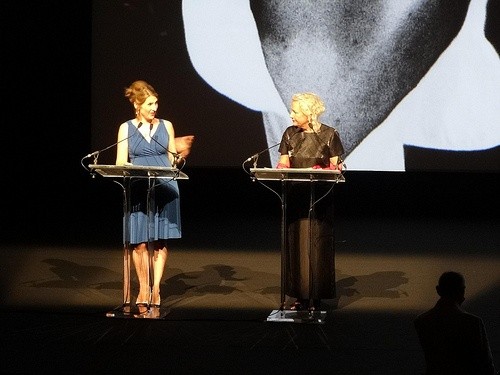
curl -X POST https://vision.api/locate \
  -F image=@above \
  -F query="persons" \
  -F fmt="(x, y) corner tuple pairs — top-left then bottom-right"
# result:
(414, 271), (497, 375)
(116, 79), (194, 307)
(276, 91), (346, 312)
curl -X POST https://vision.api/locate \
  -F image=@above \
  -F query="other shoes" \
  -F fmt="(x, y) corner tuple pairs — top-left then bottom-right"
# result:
(151, 289), (160, 305)
(136, 287), (151, 305)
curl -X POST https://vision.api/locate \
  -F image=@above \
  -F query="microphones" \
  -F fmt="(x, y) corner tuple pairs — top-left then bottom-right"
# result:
(149, 124), (185, 160)
(83, 122), (143, 158)
(247, 126), (301, 161)
(308, 122), (350, 169)
